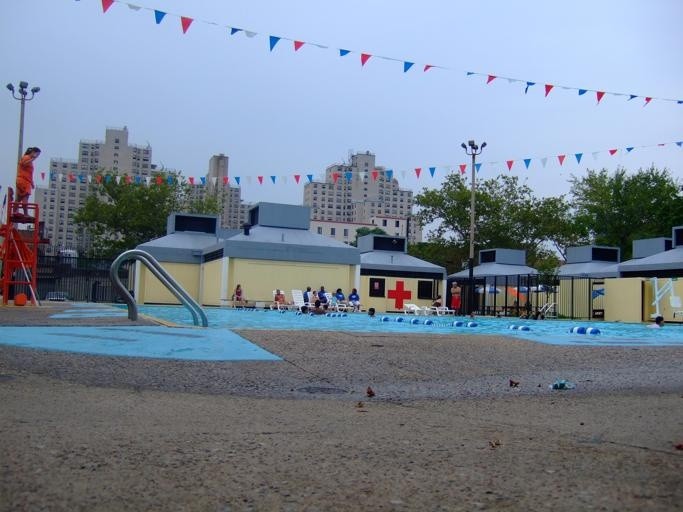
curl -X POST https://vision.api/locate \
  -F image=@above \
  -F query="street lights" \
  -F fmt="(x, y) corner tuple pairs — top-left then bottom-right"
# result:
(6, 80), (40, 230)
(460, 139), (486, 313)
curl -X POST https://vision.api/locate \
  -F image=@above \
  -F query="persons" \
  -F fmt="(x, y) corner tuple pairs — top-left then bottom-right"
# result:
(648, 316), (664, 330)
(274, 289), (286, 311)
(431, 296), (443, 314)
(13, 146), (40, 221)
(299, 285), (360, 315)
(450, 281), (461, 316)
(366, 307), (375, 318)
(232, 284), (243, 310)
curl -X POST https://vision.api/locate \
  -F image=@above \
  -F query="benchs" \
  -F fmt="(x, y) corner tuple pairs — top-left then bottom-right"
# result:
(220, 298), (272, 308)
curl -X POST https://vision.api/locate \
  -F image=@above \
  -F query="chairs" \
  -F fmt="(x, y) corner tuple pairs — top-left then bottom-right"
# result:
(273, 289), (455, 315)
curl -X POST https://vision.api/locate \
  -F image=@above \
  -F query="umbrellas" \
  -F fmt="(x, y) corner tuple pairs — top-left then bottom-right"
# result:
(529, 284), (550, 293)
(512, 285), (529, 293)
(506, 287), (526, 307)
(475, 284), (501, 306)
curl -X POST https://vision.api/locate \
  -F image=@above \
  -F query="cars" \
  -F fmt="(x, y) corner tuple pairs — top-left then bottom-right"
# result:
(45, 291), (65, 302)
